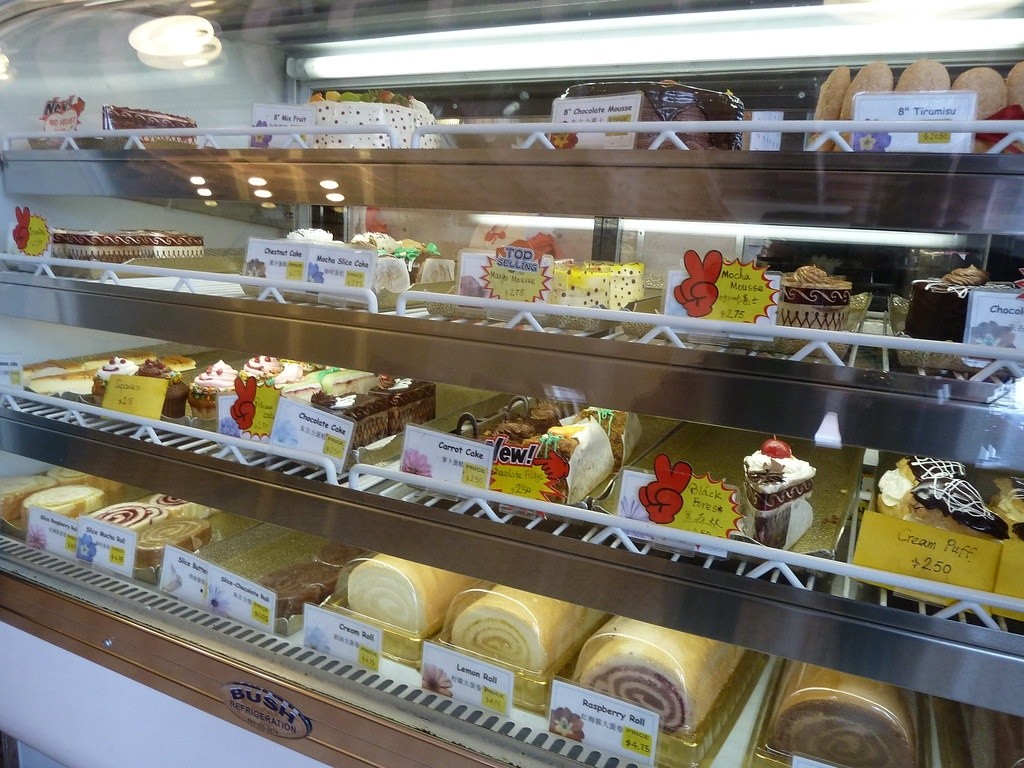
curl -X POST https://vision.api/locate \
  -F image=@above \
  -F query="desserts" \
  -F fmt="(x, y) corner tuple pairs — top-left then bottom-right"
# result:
(0, 351), (1024, 768)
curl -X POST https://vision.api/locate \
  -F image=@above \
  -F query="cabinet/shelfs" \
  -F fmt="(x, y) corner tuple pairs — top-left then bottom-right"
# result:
(0, 1), (1024, 768)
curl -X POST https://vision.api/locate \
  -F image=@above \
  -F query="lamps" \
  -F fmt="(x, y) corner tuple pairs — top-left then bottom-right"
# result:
(285, 8), (1023, 88)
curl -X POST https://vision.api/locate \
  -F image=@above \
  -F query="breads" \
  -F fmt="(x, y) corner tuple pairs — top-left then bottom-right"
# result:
(806, 60), (1024, 154)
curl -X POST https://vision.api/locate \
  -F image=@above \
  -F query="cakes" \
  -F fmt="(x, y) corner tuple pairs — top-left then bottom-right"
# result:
(767, 263), (852, 334)
(554, 80), (746, 152)
(45, 226), (204, 278)
(898, 264), (1024, 344)
(284, 227), (456, 293)
(296, 89), (439, 150)
(548, 249), (643, 315)
